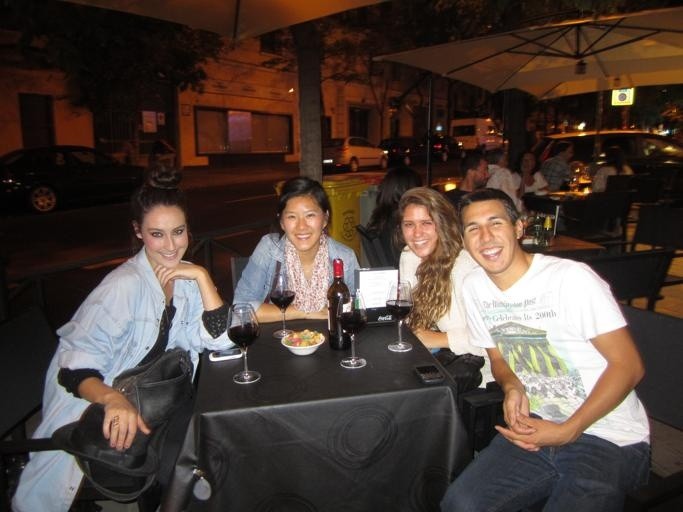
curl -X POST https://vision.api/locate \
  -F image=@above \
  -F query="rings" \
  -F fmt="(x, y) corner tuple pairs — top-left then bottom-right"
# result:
(112, 414), (120, 430)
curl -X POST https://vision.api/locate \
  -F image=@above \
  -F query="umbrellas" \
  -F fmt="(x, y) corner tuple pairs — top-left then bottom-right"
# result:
(371, 1), (683, 169)
(66, 1), (383, 45)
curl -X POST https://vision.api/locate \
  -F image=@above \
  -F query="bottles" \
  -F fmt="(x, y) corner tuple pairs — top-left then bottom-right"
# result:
(326, 258), (351, 352)
(520, 208), (554, 252)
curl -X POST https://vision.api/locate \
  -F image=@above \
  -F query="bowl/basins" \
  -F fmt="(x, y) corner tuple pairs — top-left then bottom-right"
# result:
(280, 334), (325, 356)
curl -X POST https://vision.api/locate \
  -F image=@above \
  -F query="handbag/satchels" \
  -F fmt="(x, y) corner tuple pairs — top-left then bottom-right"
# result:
(54, 347), (193, 503)
(433, 348), (485, 394)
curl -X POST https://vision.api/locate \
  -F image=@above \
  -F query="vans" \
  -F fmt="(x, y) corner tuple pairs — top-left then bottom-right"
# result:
(449, 117), (505, 154)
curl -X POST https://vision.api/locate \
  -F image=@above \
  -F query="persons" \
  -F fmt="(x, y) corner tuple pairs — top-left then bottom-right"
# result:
(395, 185), (463, 354)
(437, 186), (653, 511)
(367, 164), (423, 268)
(232, 175), (361, 324)
(458, 127), (636, 222)
(8, 182), (241, 512)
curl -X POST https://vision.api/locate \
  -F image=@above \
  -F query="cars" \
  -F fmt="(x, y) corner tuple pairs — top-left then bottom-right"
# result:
(428, 134), (466, 160)
(531, 129), (683, 169)
(0, 144), (141, 210)
(381, 135), (448, 164)
(322, 136), (389, 172)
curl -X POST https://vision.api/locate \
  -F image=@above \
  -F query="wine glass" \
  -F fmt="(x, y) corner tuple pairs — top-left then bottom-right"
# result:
(385, 279), (414, 352)
(335, 292), (368, 370)
(270, 273), (296, 339)
(227, 302), (261, 384)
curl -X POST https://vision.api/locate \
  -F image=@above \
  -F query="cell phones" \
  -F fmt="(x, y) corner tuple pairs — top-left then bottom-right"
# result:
(414, 363), (445, 384)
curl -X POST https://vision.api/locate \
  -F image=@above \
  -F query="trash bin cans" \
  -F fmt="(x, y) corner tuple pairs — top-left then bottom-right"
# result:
(272, 174), (377, 251)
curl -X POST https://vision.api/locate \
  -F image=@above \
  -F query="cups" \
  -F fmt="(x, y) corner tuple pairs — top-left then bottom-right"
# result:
(569, 178), (579, 192)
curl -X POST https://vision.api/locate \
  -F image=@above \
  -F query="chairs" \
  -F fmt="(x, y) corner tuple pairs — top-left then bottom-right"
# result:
(520, 193), (557, 213)
(1, 274), (163, 511)
(464, 304), (682, 506)
(355, 224), (388, 267)
(230, 256), (250, 290)
(560, 173), (683, 311)
